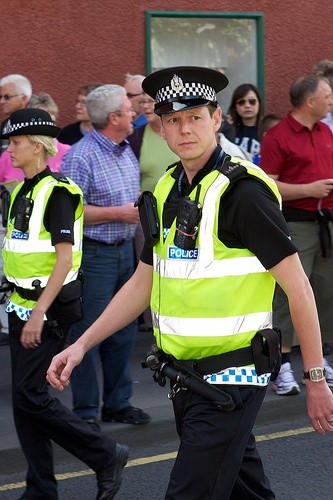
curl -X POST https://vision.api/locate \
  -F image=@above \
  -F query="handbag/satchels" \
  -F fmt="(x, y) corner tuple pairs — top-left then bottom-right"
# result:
(49, 279), (83, 327)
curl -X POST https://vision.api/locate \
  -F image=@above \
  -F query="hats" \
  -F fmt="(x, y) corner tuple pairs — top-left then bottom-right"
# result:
(142, 66), (230, 118)
(0, 108), (62, 140)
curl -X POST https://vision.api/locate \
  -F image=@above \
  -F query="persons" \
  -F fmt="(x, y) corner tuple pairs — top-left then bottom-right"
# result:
(0, 108), (132, 500)
(220, 83), (262, 166)
(62, 85), (152, 428)
(0, 73), (32, 156)
(62, 84), (102, 146)
(48, 65), (333, 500)
(261, 75), (333, 394)
(124, 73), (148, 129)
(312, 60), (333, 134)
(0, 93), (71, 185)
(129, 91), (180, 331)
(252, 114), (281, 166)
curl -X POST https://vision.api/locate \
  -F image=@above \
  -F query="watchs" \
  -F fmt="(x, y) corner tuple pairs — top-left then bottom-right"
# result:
(303, 367), (327, 383)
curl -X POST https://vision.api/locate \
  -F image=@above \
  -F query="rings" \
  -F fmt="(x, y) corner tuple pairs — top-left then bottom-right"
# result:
(328, 415), (333, 422)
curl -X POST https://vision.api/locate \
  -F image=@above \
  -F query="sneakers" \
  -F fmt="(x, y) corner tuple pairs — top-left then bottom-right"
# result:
(302, 358), (333, 387)
(270, 362), (301, 395)
(96, 443), (130, 499)
(101, 405), (151, 423)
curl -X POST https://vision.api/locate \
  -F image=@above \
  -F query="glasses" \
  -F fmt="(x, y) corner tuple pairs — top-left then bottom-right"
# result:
(236, 99), (256, 107)
(0, 94), (18, 100)
(127, 92), (141, 98)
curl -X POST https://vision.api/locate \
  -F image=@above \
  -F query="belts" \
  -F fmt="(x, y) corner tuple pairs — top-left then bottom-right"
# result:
(195, 347), (253, 375)
(99, 240), (124, 246)
(11, 287), (46, 301)
